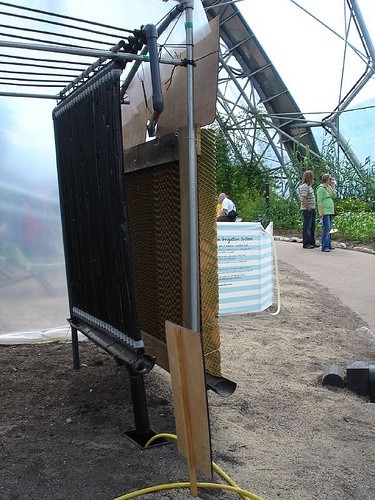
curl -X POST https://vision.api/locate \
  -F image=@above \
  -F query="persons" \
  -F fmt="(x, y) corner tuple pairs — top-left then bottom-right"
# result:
(216, 193), (236, 222)
(316, 173), (337, 252)
(300, 170), (320, 249)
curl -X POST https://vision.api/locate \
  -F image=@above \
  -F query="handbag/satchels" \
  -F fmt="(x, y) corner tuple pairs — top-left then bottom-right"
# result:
(228, 210), (238, 219)
(334, 210), (337, 216)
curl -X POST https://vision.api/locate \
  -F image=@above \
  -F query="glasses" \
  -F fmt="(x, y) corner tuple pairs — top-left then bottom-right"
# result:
(329, 178), (332, 180)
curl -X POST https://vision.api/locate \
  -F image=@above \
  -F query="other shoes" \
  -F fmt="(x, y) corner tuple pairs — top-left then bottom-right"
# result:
(330, 246), (335, 249)
(313, 243), (320, 246)
(323, 248), (330, 252)
(305, 245), (314, 249)
